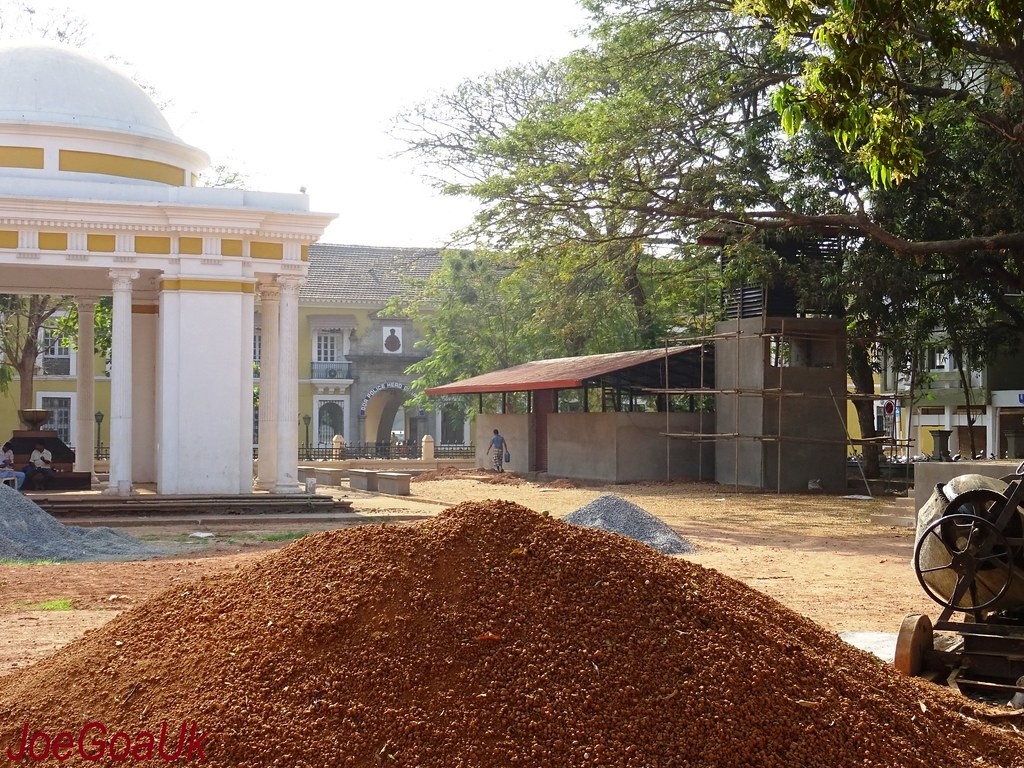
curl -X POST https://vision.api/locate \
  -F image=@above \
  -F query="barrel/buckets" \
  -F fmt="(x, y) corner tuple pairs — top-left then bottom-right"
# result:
(504, 452), (510, 462)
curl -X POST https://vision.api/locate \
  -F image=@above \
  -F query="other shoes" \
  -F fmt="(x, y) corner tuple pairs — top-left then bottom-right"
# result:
(501, 469), (504, 473)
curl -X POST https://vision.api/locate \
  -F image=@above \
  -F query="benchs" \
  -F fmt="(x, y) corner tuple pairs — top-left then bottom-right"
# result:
(12, 462), (91, 488)
(313, 468), (343, 487)
(375, 472), (412, 495)
(297, 465), (314, 483)
(347, 468), (379, 491)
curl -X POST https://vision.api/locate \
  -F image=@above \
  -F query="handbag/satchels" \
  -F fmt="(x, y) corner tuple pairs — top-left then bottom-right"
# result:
(505, 452), (510, 462)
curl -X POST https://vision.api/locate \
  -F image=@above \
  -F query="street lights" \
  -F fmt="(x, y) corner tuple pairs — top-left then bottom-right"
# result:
(95, 411), (105, 461)
(303, 414), (311, 459)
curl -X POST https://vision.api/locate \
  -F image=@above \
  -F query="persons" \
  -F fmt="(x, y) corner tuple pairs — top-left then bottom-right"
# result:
(0, 442), (26, 490)
(389, 432), (399, 458)
(24, 437), (54, 493)
(485, 429), (510, 472)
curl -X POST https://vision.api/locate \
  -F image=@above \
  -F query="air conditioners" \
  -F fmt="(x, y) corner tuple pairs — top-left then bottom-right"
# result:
(325, 367), (344, 378)
